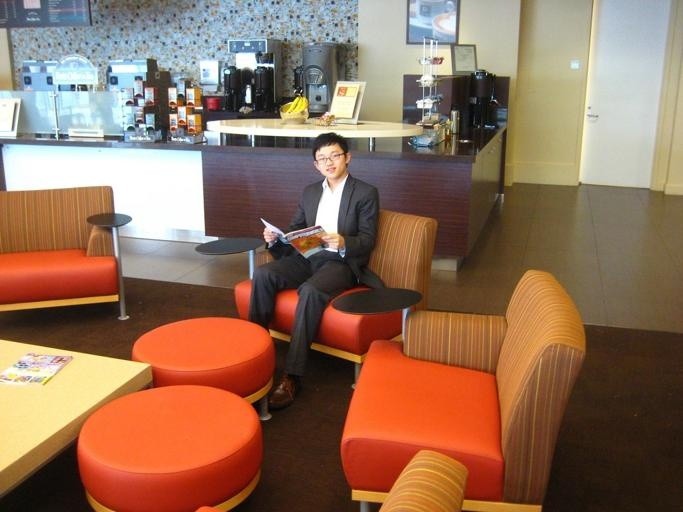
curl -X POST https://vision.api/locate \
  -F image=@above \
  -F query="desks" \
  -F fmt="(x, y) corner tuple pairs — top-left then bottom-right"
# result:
(0, 337), (153, 503)
(194, 235), (266, 278)
(86, 211), (131, 321)
(204, 117), (425, 152)
(330, 286), (423, 343)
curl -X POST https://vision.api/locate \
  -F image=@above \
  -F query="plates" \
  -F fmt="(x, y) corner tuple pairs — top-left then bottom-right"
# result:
(416, 57), (444, 65)
(413, 101), (437, 109)
(415, 80), (442, 87)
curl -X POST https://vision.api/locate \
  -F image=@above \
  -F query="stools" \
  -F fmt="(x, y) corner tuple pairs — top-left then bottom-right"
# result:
(74, 383), (263, 512)
(130, 315), (277, 423)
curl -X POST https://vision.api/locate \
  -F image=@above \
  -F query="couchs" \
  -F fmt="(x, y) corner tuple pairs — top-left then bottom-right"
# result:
(0, 184), (132, 321)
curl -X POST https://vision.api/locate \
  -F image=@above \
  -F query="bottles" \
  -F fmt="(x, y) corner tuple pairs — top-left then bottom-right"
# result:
(450, 105), (460, 134)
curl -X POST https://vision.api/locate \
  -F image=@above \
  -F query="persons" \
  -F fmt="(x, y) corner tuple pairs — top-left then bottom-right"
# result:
(248, 132), (380, 410)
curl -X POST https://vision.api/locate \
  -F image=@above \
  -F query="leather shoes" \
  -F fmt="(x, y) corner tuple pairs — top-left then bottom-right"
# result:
(267, 371), (302, 410)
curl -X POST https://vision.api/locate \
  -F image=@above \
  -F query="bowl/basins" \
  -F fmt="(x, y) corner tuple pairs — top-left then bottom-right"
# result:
(278, 103), (307, 124)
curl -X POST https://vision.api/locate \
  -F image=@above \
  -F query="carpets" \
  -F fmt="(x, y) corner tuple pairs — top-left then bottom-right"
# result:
(0, 274), (681, 512)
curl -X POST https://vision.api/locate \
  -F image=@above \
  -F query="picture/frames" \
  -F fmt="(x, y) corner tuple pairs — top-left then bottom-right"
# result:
(405, 0), (459, 44)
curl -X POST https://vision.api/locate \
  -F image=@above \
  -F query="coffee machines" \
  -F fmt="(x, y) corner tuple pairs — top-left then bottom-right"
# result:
(222, 38), (281, 116)
(469, 70), (497, 129)
(301, 41), (346, 114)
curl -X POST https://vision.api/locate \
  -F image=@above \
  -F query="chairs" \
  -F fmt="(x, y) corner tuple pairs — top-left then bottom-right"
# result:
(231, 206), (438, 388)
(380, 448), (471, 512)
(339, 266), (586, 512)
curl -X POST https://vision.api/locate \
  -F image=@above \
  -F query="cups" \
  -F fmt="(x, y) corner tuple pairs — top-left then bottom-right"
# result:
(206, 96), (221, 110)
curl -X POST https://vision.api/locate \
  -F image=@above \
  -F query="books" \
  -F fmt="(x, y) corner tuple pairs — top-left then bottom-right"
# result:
(330, 84), (360, 118)
(0, 352), (73, 387)
(258, 215), (330, 259)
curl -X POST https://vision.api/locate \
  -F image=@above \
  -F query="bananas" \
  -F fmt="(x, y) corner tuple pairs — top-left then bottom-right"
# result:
(286, 92), (308, 115)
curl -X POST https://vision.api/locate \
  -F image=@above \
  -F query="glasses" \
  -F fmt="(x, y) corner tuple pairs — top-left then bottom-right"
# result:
(316, 152), (346, 166)
